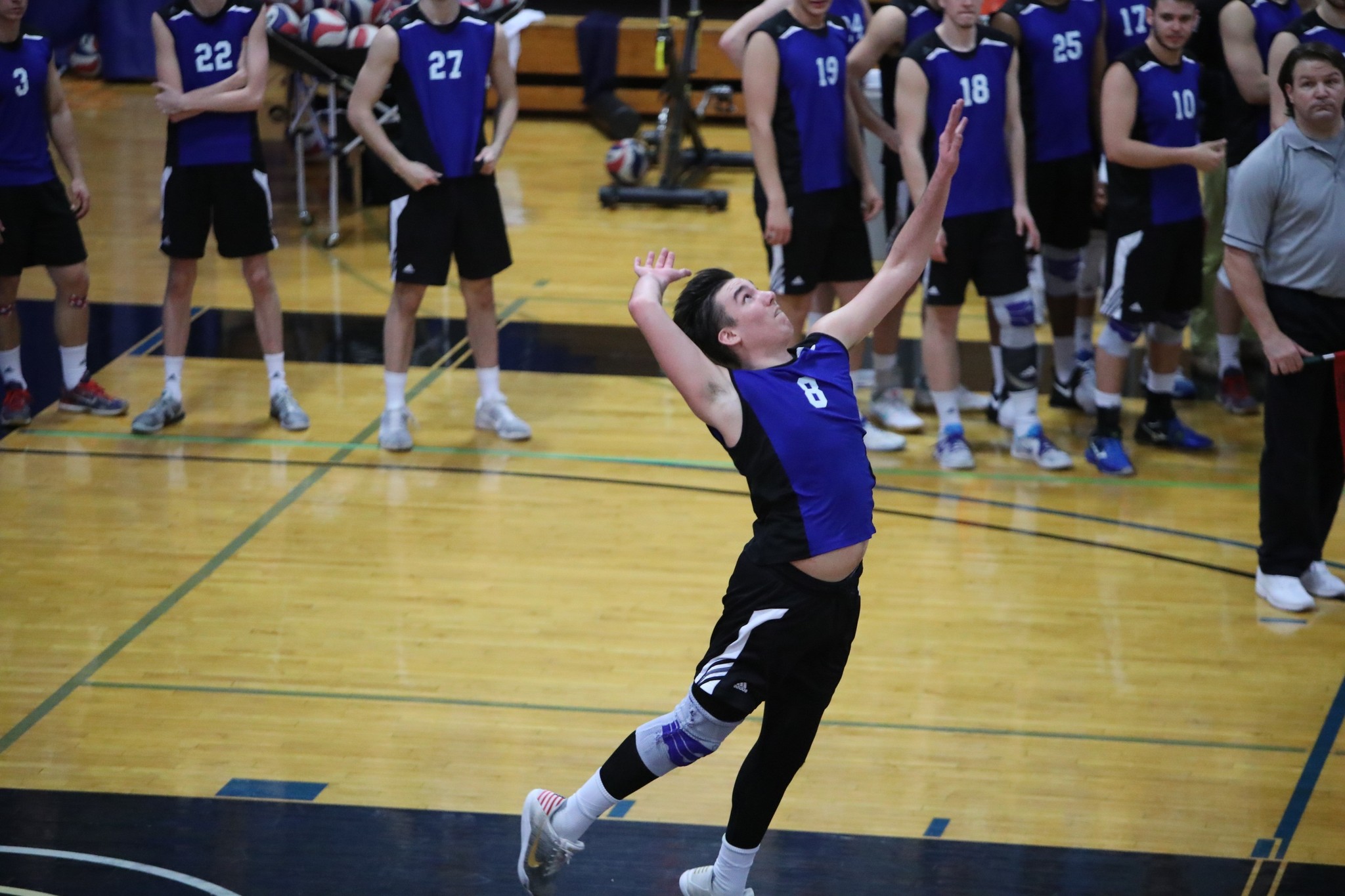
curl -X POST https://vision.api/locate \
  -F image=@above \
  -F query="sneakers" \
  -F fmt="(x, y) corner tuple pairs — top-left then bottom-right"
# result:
(473, 394), (532, 441)
(1133, 404), (1216, 452)
(1304, 558), (1345, 601)
(1083, 426), (1136, 476)
(57, 369), (129, 415)
(269, 384), (311, 429)
(378, 405), (417, 451)
(517, 787), (586, 896)
(132, 390), (186, 432)
(1252, 568), (1316, 613)
(1, 375), (33, 424)
(931, 422), (977, 471)
(1005, 421), (1073, 470)
(678, 865), (755, 896)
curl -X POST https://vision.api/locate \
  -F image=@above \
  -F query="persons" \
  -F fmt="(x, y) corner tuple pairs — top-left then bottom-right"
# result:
(520, 98), (967, 896)
(0, 0), (1345, 614)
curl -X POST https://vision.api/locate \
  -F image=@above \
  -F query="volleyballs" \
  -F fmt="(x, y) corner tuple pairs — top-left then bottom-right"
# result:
(604, 137), (651, 185)
(264, 0), (518, 54)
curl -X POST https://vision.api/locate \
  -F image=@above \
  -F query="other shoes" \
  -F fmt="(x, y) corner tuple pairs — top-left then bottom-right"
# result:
(1216, 364), (1257, 418)
(868, 385), (925, 432)
(911, 373), (991, 411)
(1073, 346), (1096, 381)
(986, 389), (1018, 428)
(1046, 366), (1091, 412)
(859, 417), (906, 453)
(1139, 359), (1193, 395)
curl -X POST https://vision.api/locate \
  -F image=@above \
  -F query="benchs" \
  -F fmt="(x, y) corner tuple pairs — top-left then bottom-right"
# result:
(487, 11), (754, 124)
